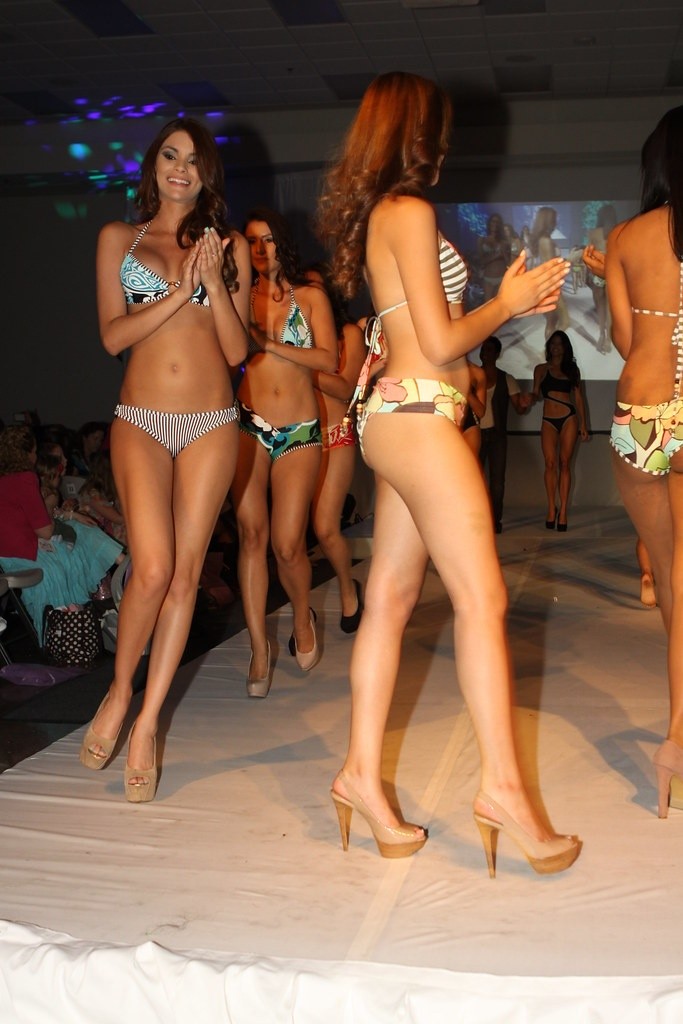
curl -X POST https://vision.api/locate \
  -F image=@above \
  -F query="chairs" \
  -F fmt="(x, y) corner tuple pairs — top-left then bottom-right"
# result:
(0, 565), (44, 666)
(58, 475), (88, 502)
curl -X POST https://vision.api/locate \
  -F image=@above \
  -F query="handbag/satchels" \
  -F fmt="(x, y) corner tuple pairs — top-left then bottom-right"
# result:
(40, 604), (103, 667)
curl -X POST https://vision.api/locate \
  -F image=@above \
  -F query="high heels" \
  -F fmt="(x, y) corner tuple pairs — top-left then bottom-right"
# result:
(124, 718), (158, 803)
(546, 504), (558, 529)
(651, 741), (683, 818)
(472, 789), (582, 881)
(332, 771), (427, 858)
(81, 692), (124, 773)
(244, 580), (364, 698)
(558, 511), (567, 532)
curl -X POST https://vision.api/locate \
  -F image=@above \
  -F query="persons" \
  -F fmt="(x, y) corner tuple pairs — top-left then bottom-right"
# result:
(455, 354), (488, 462)
(230, 212), (340, 701)
(308, 69), (585, 882)
(0, 406), (359, 664)
(518, 328), (595, 535)
(581, 106), (683, 819)
(280, 264), (378, 660)
(458, 203), (621, 364)
(465, 334), (528, 536)
(74, 116), (254, 804)
